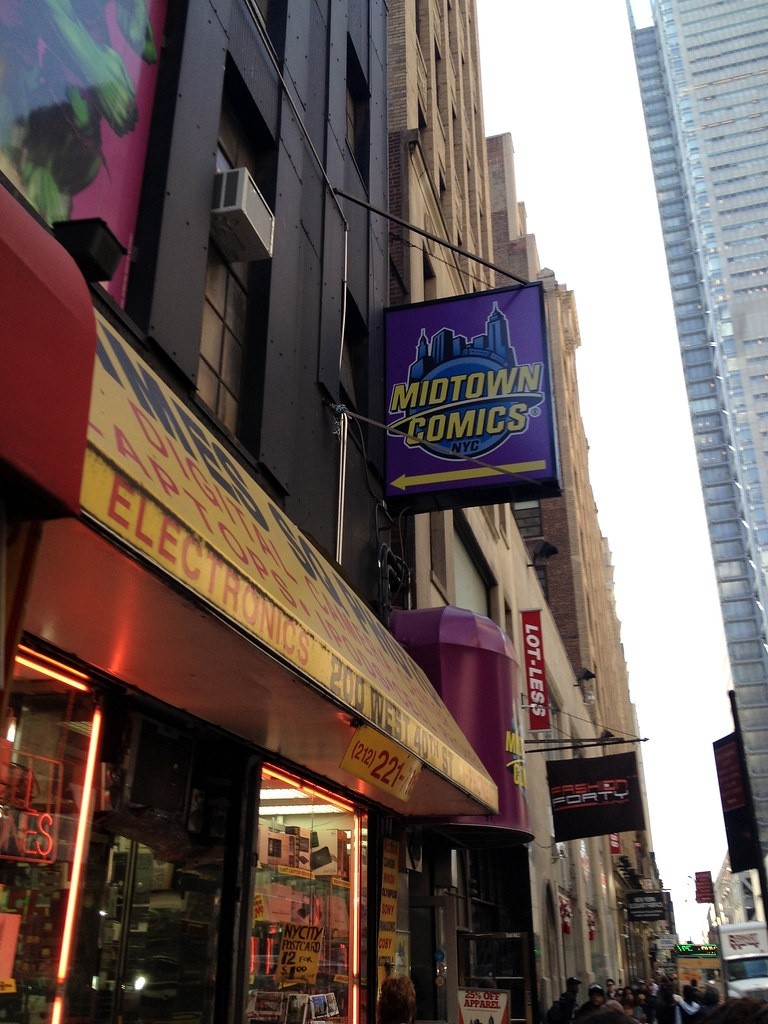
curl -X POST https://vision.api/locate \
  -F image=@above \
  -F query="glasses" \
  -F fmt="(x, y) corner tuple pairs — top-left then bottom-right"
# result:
(607, 984), (614, 986)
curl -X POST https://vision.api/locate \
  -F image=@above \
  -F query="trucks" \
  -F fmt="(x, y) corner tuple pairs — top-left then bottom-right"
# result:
(709, 922), (768, 1003)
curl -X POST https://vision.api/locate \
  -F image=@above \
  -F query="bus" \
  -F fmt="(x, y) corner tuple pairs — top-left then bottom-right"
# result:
(675, 942), (726, 1008)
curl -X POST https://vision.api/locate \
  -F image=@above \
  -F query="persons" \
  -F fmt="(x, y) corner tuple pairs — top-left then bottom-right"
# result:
(376, 973), (417, 1024)
(546, 969), (768, 1024)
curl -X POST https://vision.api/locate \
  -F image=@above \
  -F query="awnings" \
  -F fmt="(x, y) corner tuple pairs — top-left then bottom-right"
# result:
(0, 182), (97, 522)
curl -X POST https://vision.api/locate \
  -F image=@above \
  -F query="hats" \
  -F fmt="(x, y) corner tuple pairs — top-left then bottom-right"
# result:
(566, 977), (583, 985)
(637, 994), (646, 1000)
(589, 984), (604, 994)
